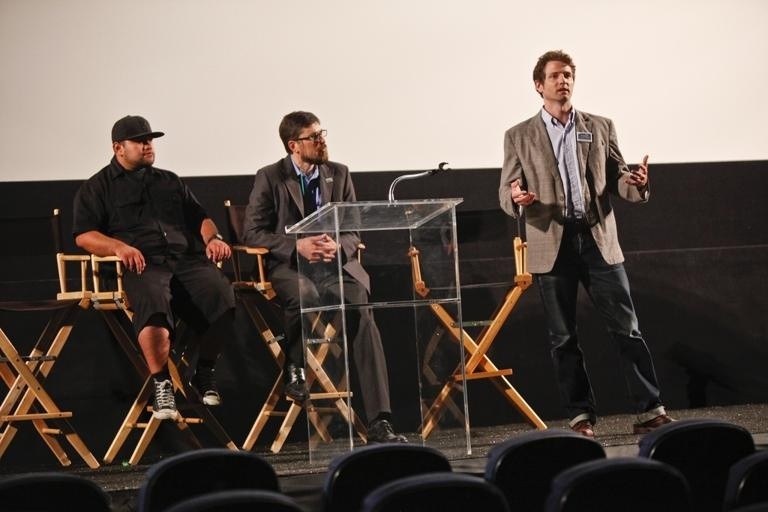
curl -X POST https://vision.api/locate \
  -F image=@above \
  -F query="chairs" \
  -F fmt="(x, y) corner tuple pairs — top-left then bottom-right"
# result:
(409, 207), (548, 439)
(222, 199), (369, 455)
(91, 253), (241, 466)
(0, 206), (100, 468)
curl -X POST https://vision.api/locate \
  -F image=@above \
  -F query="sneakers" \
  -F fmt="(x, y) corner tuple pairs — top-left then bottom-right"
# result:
(188, 367), (221, 407)
(152, 378), (178, 420)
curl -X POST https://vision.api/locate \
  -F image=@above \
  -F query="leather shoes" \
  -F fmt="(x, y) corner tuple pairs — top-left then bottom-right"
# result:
(283, 359), (311, 401)
(572, 420), (595, 441)
(633, 414), (680, 435)
(366, 419), (408, 445)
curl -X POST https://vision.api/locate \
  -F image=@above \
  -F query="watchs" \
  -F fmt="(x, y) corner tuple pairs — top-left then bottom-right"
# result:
(208, 233), (223, 243)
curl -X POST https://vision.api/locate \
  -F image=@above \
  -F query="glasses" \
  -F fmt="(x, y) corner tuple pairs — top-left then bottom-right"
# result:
(290, 130), (328, 143)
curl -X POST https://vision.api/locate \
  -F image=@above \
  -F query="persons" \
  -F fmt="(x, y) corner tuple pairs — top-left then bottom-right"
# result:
(72, 115), (236, 421)
(241, 111), (408, 445)
(498, 51), (678, 441)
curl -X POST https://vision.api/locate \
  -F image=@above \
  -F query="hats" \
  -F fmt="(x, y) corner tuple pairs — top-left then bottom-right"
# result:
(112, 115), (165, 142)
